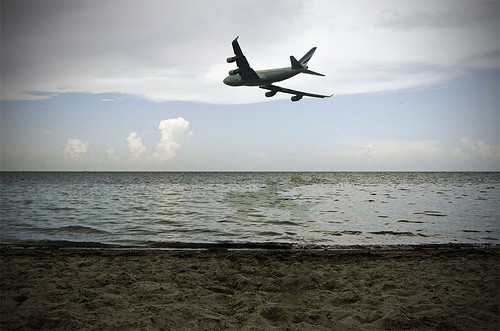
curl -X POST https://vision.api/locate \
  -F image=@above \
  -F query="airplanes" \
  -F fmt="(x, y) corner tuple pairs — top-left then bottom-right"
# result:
(223, 36), (334, 101)
